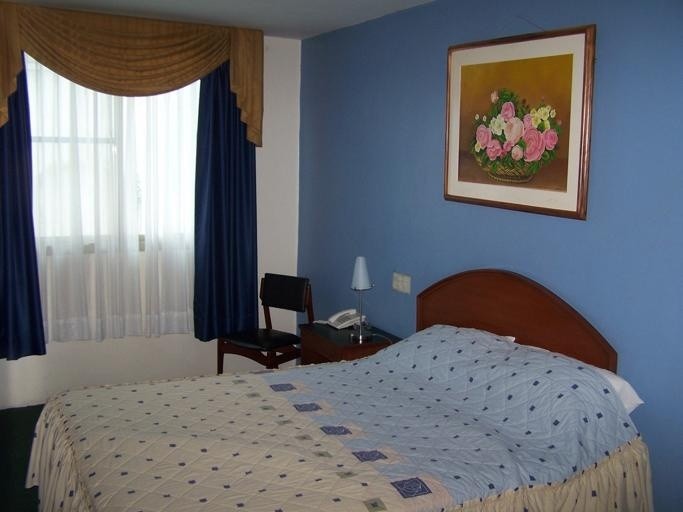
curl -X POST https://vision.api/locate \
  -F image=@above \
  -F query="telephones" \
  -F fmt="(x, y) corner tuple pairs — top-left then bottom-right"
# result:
(328, 308), (366, 329)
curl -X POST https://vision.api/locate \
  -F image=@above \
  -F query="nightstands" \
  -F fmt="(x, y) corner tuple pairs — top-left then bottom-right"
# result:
(299, 324), (403, 365)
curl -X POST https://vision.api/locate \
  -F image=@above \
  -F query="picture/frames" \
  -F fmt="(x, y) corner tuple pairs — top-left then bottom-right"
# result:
(444, 25), (596, 220)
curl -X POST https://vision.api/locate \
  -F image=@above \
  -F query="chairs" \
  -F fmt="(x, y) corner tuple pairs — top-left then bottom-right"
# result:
(217, 273), (315, 374)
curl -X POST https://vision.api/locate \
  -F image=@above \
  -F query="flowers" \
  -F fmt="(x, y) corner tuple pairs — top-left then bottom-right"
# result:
(470, 89), (562, 177)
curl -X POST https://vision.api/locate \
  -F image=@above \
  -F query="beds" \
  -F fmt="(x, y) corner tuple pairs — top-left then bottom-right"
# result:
(25, 268), (653, 512)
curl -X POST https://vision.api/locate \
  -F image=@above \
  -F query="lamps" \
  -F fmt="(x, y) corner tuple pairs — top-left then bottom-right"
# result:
(350, 256), (376, 345)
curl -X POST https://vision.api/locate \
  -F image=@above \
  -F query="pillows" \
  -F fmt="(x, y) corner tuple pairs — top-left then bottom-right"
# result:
(502, 334), (645, 416)
